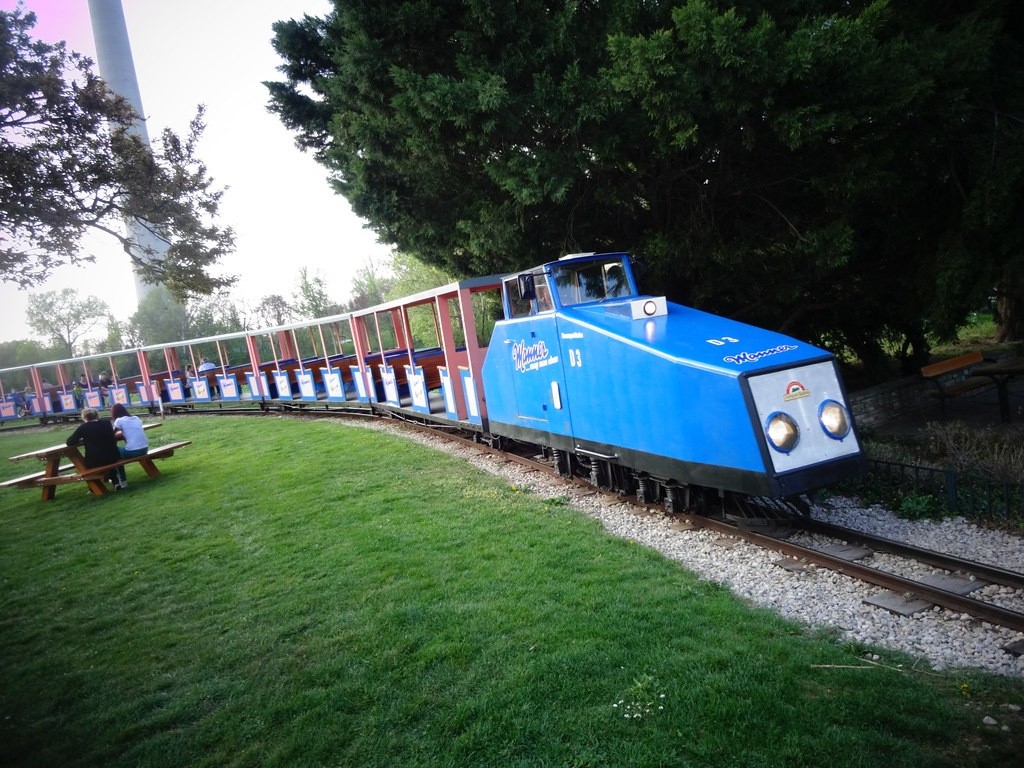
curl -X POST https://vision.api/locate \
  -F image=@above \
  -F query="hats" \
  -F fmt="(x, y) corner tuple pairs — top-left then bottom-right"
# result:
(101, 371), (109, 376)
(202, 357), (209, 362)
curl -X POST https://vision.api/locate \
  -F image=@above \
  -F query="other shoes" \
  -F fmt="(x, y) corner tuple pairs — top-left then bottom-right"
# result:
(114, 484), (121, 491)
(119, 480), (128, 488)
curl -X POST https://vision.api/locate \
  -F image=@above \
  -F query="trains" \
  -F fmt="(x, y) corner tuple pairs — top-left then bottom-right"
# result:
(0, 254), (861, 524)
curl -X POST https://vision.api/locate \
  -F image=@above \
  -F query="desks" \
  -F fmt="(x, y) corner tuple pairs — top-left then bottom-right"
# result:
(9, 423), (164, 497)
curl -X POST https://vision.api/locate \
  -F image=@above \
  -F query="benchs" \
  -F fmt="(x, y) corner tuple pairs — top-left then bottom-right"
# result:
(0, 463), (75, 488)
(0, 378), (115, 421)
(117, 362), (255, 407)
(63, 441), (193, 480)
(256, 345), (466, 404)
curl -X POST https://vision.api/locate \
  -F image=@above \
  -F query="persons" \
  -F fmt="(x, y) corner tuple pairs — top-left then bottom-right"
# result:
(41, 378), (53, 389)
(72, 381), (85, 410)
(78, 373), (89, 389)
(66, 408), (121, 495)
(184, 364), (196, 388)
(7, 387), (31, 418)
(108, 402), (149, 489)
(98, 371), (114, 397)
(24, 387), (37, 415)
(198, 357), (216, 372)
(529, 281), (554, 316)
